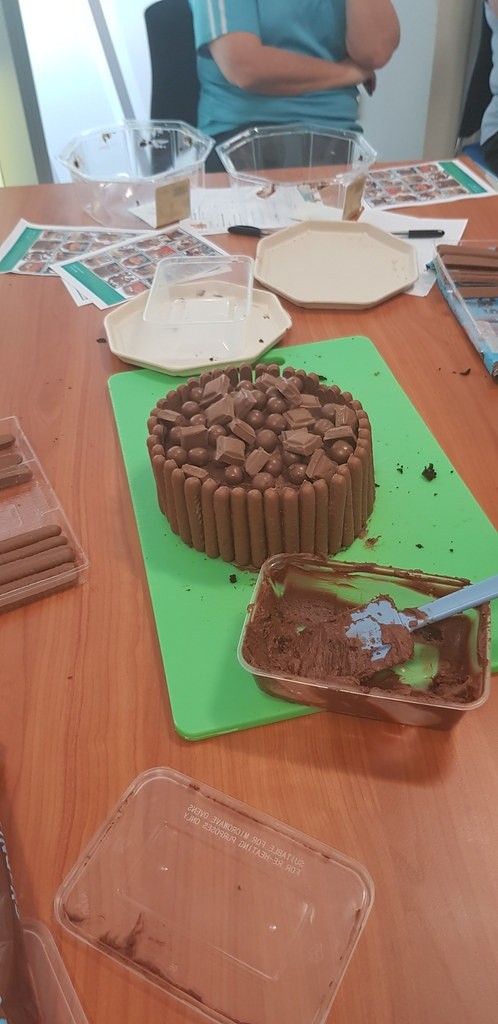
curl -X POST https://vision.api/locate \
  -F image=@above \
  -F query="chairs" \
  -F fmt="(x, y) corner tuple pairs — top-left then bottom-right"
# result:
(143, 0), (199, 172)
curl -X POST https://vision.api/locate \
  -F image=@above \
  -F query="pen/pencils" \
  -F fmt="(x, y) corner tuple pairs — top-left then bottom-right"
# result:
(390, 229), (446, 238)
(228, 225), (272, 237)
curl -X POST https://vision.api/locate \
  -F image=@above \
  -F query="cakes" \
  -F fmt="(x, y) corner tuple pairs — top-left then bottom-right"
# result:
(149, 364), (377, 572)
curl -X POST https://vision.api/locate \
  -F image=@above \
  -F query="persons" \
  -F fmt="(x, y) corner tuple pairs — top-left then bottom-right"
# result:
(186, 0), (401, 176)
(480, 0), (498, 177)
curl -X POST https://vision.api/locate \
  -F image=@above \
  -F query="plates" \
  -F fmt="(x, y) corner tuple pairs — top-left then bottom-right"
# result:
(252, 222), (419, 313)
(102, 280), (290, 377)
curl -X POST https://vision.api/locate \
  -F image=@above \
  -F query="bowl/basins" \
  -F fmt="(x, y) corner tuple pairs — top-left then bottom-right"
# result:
(140, 255), (263, 360)
(235, 549), (495, 731)
(57, 115), (218, 234)
(212, 121), (381, 230)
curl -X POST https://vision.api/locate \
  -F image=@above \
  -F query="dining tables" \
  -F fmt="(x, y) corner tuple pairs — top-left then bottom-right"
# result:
(0, 156), (498, 1024)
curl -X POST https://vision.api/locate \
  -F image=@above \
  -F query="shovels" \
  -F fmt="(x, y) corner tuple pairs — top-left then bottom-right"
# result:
(300, 572), (498, 681)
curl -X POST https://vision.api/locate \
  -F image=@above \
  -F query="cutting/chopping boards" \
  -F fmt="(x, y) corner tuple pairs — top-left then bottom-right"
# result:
(106, 333), (497, 741)
